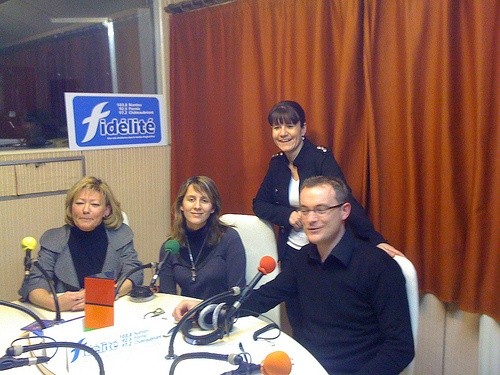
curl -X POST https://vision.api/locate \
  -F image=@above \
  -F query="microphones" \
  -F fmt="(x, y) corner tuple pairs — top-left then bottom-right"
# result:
(20, 236), (37, 280)
(168, 286), (241, 357)
(0, 357), (48, 371)
(115, 261), (157, 298)
(221, 350), (292, 375)
(169, 352), (243, 374)
(226, 255), (276, 319)
(6, 342), (104, 375)
(151, 240), (180, 285)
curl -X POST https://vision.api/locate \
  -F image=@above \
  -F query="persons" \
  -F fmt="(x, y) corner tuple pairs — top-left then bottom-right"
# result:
(18, 176), (145, 311)
(252, 100), (407, 341)
(171, 175), (415, 375)
(159, 177), (246, 304)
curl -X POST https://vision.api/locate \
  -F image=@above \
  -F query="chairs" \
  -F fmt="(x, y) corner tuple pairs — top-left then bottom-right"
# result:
(220, 213), (281, 331)
(376, 243), (419, 375)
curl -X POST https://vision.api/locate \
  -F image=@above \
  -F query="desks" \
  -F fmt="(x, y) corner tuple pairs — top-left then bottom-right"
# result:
(0, 290), (330, 375)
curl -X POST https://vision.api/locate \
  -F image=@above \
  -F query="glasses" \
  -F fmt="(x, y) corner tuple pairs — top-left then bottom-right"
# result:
(296, 202), (344, 215)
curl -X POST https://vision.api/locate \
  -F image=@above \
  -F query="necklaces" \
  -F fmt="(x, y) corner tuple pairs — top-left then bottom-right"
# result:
(184, 232), (208, 281)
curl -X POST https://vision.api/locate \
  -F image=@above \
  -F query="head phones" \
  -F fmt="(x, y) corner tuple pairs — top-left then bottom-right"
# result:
(182, 303), (233, 346)
